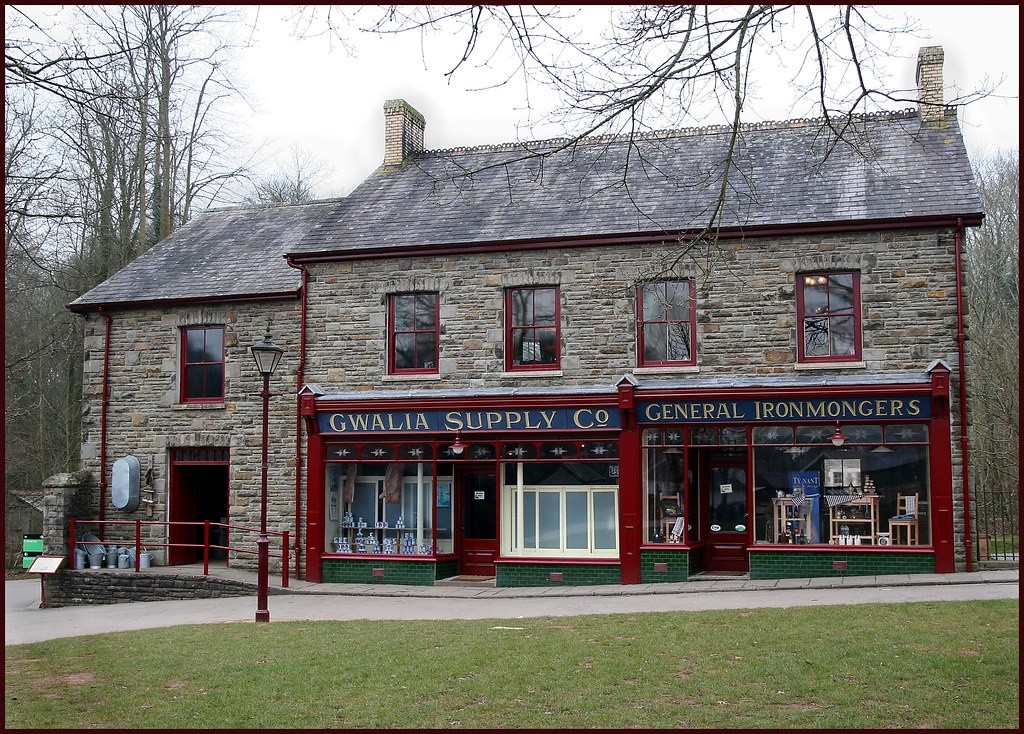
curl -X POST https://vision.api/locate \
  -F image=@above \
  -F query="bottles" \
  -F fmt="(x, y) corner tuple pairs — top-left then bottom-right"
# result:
(848, 480), (853, 496)
(781, 526), (805, 544)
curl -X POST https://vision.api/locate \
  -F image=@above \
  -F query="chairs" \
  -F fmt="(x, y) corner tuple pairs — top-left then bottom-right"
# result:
(888, 493), (919, 546)
(659, 492), (685, 544)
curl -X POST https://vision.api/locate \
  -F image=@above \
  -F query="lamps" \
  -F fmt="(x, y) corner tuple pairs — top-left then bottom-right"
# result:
(783, 447), (806, 453)
(870, 446), (894, 452)
(661, 429), (683, 453)
(448, 429), (468, 454)
(827, 421), (849, 446)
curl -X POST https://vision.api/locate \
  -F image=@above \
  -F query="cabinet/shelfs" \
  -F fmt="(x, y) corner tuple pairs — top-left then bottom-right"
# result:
(330, 526), (451, 554)
(772, 498), (813, 544)
(823, 495), (885, 545)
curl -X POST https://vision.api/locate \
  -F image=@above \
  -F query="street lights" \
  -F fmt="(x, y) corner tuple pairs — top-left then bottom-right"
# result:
(250, 317), (288, 623)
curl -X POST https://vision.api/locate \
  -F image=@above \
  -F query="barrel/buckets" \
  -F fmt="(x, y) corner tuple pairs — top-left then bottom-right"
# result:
(75, 549), (152, 568)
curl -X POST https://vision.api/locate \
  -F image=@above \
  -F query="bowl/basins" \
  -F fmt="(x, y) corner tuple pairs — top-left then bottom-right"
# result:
(79, 533), (106, 562)
